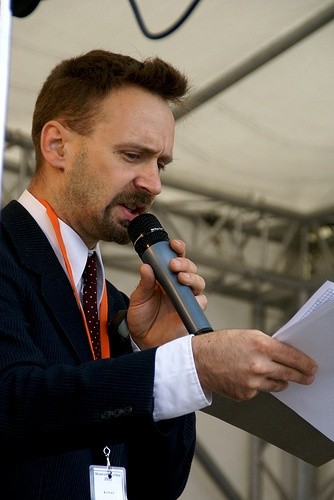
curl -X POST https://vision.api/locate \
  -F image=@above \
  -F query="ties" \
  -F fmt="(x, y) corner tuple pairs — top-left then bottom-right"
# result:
(83, 256), (102, 360)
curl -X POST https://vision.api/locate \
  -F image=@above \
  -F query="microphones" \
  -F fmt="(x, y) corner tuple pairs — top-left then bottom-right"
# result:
(127, 213), (215, 336)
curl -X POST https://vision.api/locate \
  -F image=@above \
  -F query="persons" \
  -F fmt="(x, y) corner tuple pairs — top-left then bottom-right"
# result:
(0, 50), (319, 500)
